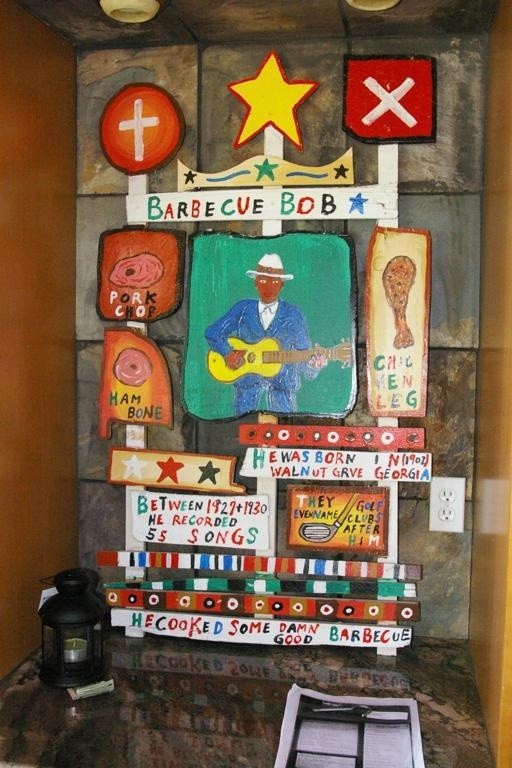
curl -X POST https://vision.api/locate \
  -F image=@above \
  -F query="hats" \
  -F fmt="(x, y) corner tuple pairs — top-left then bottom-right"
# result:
(247, 253), (294, 280)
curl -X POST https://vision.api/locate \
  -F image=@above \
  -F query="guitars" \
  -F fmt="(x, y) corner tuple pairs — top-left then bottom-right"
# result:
(209, 337), (353, 383)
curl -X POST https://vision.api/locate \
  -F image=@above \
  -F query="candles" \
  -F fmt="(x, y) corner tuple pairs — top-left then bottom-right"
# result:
(63, 637), (87, 663)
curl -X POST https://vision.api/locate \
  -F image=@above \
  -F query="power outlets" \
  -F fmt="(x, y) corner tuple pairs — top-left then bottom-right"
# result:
(428, 476), (466, 533)
